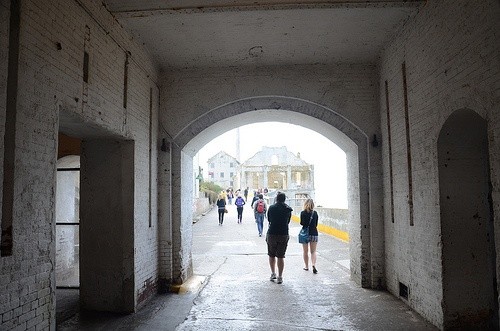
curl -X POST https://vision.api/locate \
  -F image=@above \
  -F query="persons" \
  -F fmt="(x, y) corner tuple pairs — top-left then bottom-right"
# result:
(218, 183), (250, 208)
(217, 195), (226, 226)
(251, 184), (288, 228)
(299, 199), (318, 272)
(235, 195), (246, 223)
(254, 195), (267, 237)
(266, 194), (293, 284)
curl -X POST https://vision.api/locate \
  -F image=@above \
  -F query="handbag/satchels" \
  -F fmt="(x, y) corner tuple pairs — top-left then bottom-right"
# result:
(298, 227), (310, 244)
(224, 209), (228, 213)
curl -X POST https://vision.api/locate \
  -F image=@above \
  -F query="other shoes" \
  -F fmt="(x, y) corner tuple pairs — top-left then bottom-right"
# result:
(238, 221), (242, 223)
(258, 232), (262, 237)
(219, 223), (222, 225)
(312, 266), (318, 273)
(303, 267), (309, 271)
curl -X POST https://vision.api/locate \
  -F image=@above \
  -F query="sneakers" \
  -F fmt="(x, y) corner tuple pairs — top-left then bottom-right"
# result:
(269, 273), (276, 281)
(277, 277), (283, 284)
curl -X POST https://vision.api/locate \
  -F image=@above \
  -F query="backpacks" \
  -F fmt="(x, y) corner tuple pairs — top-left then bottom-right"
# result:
(257, 200), (265, 212)
